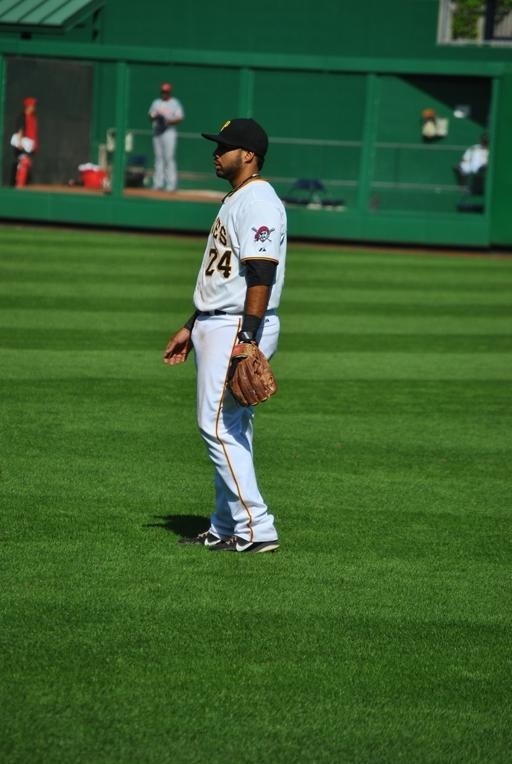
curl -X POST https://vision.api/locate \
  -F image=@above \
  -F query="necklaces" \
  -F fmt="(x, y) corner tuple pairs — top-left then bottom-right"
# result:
(221, 173), (260, 202)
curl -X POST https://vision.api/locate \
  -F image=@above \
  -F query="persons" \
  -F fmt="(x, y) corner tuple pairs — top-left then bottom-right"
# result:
(459, 132), (489, 184)
(162, 118), (279, 554)
(147, 83), (184, 193)
(9, 96), (39, 188)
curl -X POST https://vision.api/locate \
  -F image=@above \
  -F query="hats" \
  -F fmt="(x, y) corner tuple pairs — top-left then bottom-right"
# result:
(202, 118), (268, 159)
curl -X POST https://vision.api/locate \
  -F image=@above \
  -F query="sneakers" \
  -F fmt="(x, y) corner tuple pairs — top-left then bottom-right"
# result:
(179, 532), (280, 552)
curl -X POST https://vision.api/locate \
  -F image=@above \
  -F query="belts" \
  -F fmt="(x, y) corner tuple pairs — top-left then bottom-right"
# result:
(203, 310), (225, 316)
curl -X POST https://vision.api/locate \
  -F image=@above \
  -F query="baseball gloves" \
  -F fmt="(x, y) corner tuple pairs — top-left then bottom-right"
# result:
(154, 114), (167, 135)
(227, 340), (276, 406)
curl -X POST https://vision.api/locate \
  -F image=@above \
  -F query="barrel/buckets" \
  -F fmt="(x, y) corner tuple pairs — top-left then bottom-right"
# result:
(80, 170), (107, 191)
